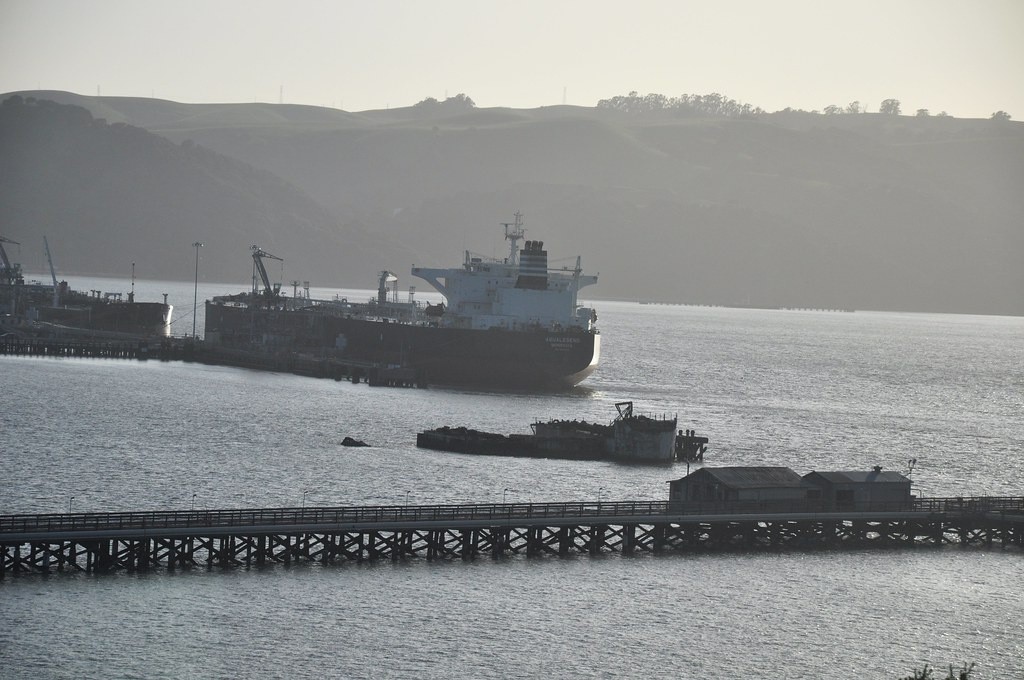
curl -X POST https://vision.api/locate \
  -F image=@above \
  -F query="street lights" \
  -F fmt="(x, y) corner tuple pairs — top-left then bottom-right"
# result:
(192, 241), (204, 339)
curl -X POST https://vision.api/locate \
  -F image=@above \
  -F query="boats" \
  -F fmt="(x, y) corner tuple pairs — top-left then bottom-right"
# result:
(0, 234), (174, 338)
(205, 211), (600, 392)
(419, 403), (710, 466)
